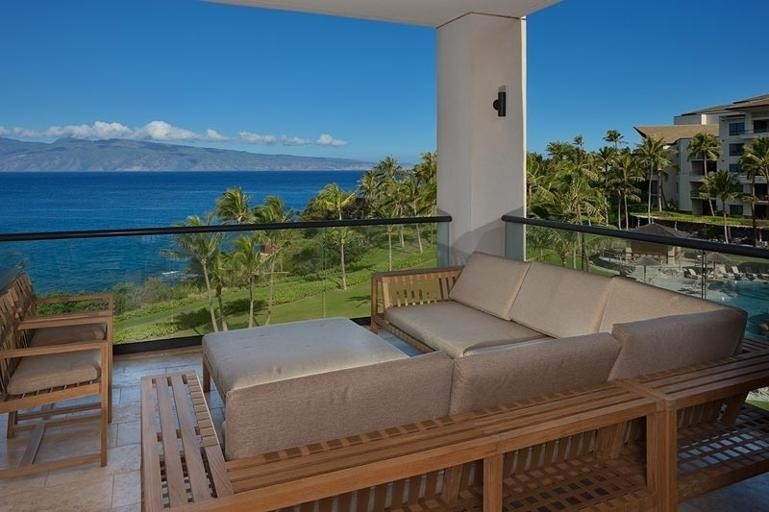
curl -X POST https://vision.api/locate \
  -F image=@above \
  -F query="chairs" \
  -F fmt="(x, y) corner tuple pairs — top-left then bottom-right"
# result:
(0, 273), (112, 481)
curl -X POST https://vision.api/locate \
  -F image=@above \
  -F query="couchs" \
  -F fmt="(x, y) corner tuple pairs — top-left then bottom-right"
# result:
(140, 249), (768, 511)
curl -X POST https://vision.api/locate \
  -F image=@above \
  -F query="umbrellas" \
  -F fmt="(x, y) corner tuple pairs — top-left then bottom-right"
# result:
(700, 252), (731, 271)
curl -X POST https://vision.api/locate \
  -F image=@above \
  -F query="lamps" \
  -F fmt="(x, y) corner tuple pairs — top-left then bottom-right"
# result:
(493, 84), (509, 116)
